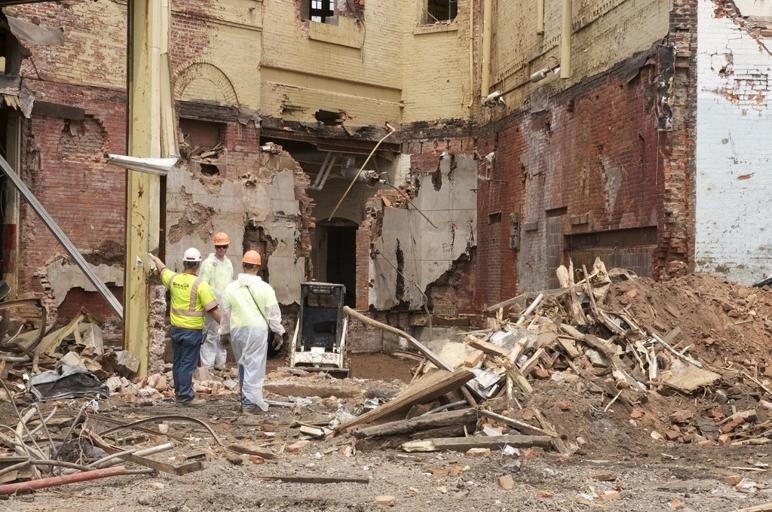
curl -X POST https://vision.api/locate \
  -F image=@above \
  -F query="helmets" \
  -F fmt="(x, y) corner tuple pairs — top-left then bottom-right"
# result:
(213, 232), (231, 245)
(241, 250), (262, 265)
(182, 247), (203, 262)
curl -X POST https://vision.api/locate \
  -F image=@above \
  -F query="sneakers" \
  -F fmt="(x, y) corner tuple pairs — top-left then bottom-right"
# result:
(208, 367), (230, 375)
(241, 397), (264, 413)
(176, 397), (206, 409)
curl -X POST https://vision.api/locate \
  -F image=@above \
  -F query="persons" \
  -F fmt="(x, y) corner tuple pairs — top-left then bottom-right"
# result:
(223, 250), (287, 414)
(197, 231), (237, 370)
(148, 247), (221, 404)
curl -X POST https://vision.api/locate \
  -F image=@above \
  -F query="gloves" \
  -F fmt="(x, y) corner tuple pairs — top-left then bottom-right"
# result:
(219, 334), (231, 349)
(149, 252), (166, 271)
(272, 332), (283, 350)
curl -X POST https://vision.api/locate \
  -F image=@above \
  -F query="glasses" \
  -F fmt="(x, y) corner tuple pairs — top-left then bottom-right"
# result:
(215, 246), (228, 249)
